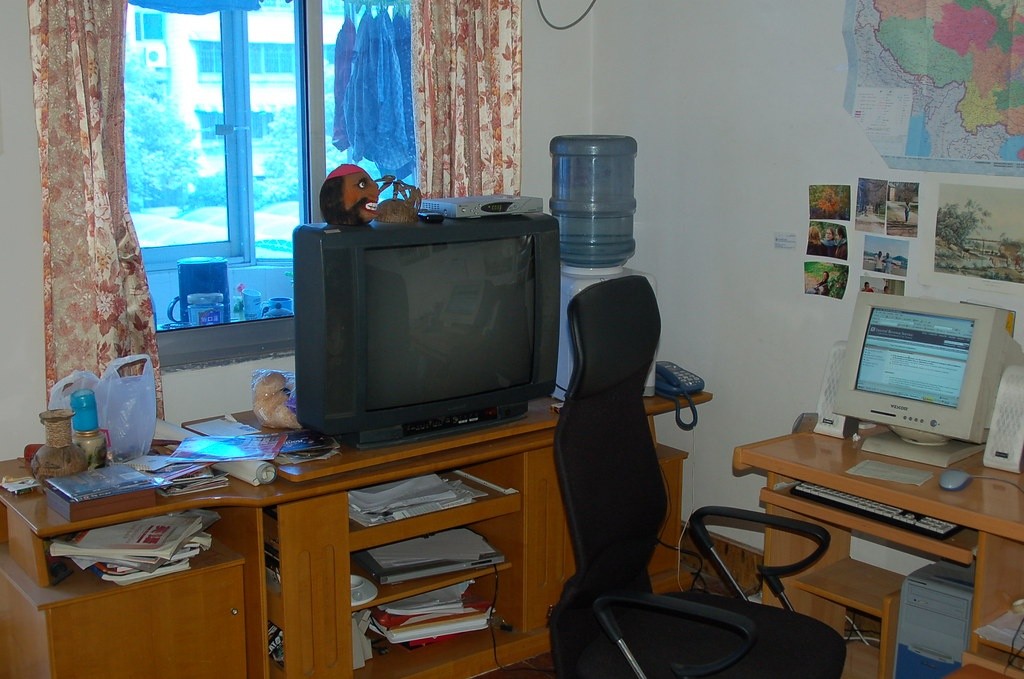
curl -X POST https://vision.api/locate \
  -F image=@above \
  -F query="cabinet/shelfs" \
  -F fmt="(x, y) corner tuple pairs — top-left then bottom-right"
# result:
(1, 390), (714, 679)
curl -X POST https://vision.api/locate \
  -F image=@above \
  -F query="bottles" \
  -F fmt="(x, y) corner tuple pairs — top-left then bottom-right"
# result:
(74, 430), (107, 471)
(188, 293), (224, 326)
(31, 409), (88, 487)
(550, 135), (636, 268)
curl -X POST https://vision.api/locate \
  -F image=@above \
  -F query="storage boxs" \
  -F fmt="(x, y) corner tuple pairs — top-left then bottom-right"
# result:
(44, 487), (156, 522)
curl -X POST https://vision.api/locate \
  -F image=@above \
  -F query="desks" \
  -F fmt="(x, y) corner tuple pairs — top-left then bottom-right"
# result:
(731, 422), (1024, 679)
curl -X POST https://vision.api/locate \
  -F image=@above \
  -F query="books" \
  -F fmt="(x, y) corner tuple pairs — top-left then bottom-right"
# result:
(50, 509), (221, 586)
(44, 432), (289, 503)
(352, 609), (373, 670)
(371, 597), (488, 643)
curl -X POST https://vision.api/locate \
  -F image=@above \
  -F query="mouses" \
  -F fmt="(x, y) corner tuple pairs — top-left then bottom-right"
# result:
(938, 468), (974, 490)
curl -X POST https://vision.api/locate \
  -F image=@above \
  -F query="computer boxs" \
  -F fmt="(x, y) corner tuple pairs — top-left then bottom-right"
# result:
(893, 560), (978, 679)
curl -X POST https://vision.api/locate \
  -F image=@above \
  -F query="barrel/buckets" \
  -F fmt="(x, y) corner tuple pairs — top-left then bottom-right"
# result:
(177, 259), (231, 327)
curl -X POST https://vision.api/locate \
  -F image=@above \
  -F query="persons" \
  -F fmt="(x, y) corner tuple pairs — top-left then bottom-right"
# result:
(875, 251), (893, 274)
(807, 271), (829, 296)
(883, 286), (889, 294)
(806, 225), (848, 260)
(862, 282), (874, 293)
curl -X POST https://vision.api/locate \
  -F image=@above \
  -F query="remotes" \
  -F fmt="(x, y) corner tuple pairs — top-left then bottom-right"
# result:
(418, 211), (445, 223)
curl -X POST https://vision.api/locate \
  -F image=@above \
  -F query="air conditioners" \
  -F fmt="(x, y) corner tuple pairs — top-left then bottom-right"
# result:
(145, 45), (167, 67)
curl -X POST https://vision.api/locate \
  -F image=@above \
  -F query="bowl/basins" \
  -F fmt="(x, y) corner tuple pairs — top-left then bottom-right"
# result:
(263, 304), (293, 319)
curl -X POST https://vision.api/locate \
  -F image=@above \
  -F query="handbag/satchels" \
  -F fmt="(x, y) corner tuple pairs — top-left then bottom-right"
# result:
(48, 353), (157, 464)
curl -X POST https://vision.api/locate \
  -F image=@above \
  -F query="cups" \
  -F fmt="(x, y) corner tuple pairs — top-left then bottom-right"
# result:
(242, 289), (261, 321)
(162, 322), (192, 330)
(261, 297), (293, 313)
(70, 389), (98, 431)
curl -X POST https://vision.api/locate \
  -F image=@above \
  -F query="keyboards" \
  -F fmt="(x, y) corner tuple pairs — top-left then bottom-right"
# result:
(794, 482), (958, 534)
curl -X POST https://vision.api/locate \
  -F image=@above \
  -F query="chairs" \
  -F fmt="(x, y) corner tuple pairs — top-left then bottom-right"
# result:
(548, 276), (847, 679)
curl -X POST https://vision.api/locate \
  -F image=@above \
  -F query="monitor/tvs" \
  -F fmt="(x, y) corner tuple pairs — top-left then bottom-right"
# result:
(293, 211), (561, 449)
(831, 291), (1024, 468)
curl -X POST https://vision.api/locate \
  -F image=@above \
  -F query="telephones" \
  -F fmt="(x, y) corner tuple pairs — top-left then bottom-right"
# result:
(656, 358), (706, 397)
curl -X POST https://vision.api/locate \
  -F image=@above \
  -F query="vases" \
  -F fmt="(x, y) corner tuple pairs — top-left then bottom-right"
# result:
(31, 408), (88, 487)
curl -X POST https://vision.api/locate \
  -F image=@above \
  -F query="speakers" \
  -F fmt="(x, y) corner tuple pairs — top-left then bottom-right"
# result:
(983, 364), (1024, 474)
(812, 340), (860, 439)
